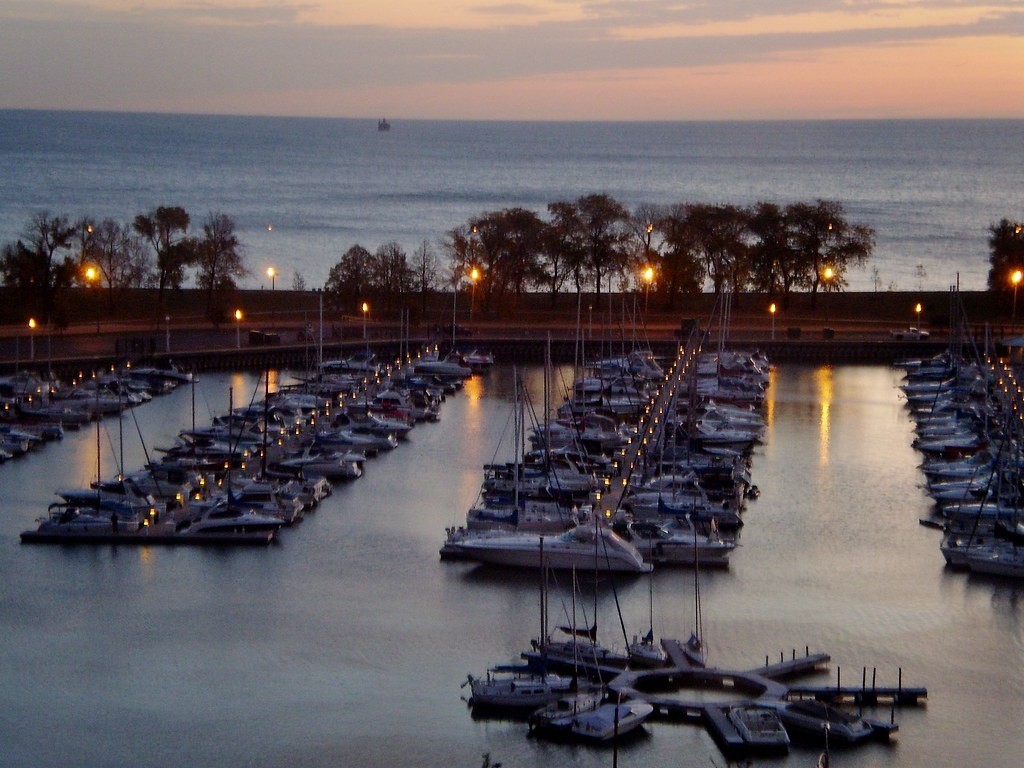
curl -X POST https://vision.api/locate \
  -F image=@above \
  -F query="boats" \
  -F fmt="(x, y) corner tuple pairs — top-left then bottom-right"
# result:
(453, 525), (654, 574)
(776, 697), (874, 743)
(529, 692), (610, 733)
(727, 703), (791, 750)
(572, 700), (655, 743)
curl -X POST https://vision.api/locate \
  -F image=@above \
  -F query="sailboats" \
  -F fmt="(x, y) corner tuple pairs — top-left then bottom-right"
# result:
(519, 513), (634, 681)
(624, 499), (707, 670)
(459, 534), (607, 710)
(0, 280), (772, 566)
(897, 268), (1023, 579)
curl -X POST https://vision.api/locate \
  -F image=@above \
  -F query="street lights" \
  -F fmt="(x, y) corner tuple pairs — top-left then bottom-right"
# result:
(1011, 270), (1022, 319)
(236, 310), (242, 349)
(362, 302), (368, 340)
(164, 314), (170, 353)
(88, 267), (100, 333)
(915, 304), (921, 330)
(470, 265), (479, 309)
(643, 267), (654, 328)
(29, 318), (35, 360)
(267, 268), (275, 296)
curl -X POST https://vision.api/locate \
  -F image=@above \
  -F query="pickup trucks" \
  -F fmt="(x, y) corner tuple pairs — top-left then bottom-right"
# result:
(891, 327), (930, 341)
